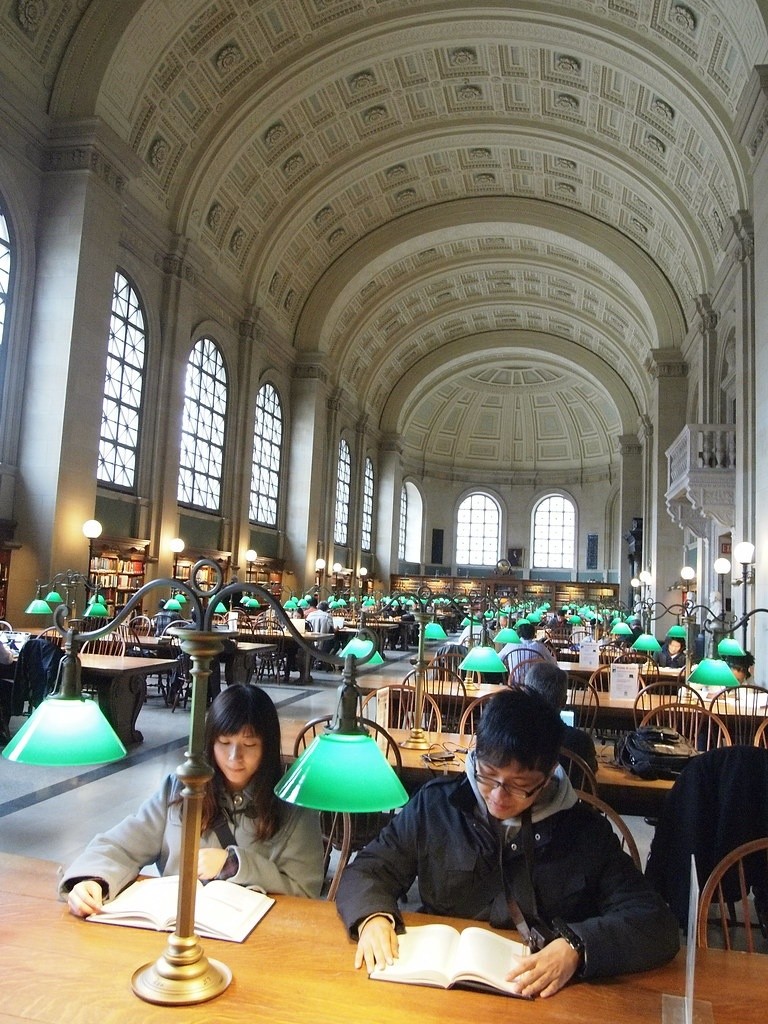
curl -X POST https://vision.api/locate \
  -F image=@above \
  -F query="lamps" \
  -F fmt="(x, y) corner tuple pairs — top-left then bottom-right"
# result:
(8, 516), (768, 812)
(1, 561), (412, 1005)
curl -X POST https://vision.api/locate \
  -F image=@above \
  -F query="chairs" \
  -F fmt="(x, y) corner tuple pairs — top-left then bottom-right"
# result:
(1, 607), (768, 955)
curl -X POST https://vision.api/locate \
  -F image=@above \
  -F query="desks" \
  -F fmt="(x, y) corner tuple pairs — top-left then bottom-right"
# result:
(16, 652), (179, 747)
(12, 627), (277, 687)
(557, 661), (691, 685)
(558, 647), (647, 662)
(231, 628), (335, 683)
(348, 673), (767, 726)
(344, 623), (397, 650)
(278, 719), (678, 800)
(2, 854), (768, 1024)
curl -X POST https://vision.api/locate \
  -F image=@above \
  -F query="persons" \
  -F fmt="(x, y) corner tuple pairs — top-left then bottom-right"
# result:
(222, 576), (244, 610)
(652, 637), (687, 668)
(58, 684), (325, 917)
(0, 641), (14, 744)
(688, 652), (755, 699)
(545, 610), (645, 650)
(461, 613), (557, 684)
(383, 603), (432, 650)
(526, 663), (599, 794)
(267, 595), (340, 682)
(151, 605), (237, 703)
(337, 684), (680, 997)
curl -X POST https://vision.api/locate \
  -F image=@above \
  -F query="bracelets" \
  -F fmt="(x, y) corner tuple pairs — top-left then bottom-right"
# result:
(554, 919), (585, 978)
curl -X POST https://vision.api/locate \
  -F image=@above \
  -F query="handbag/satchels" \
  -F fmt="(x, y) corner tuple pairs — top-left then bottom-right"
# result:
(615, 725), (701, 780)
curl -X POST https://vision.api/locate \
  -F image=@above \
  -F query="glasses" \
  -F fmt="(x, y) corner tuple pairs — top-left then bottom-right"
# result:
(473, 751), (550, 799)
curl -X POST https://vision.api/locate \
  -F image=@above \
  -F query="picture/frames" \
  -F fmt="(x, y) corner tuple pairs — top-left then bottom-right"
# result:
(506, 546), (524, 571)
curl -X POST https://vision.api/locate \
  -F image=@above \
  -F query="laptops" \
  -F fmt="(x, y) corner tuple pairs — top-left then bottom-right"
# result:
(333, 617), (344, 629)
(0, 631), (31, 658)
(559, 711), (575, 727)
(285, 619), (306, 634)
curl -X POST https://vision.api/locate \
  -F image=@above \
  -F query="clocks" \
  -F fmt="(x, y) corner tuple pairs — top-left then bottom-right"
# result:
(494, 559), (512, 576)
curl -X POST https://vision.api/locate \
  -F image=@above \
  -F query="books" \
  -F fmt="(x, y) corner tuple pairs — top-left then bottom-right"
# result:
(89, 556), (143, 624)
(391, 579), (518, 601)
(368, 924), (536, 1003)
(173, 566), (225, 600)
(85, 875), (276, 943)
(245, 573), (281, 604)
(526, 585), (615, 607)
